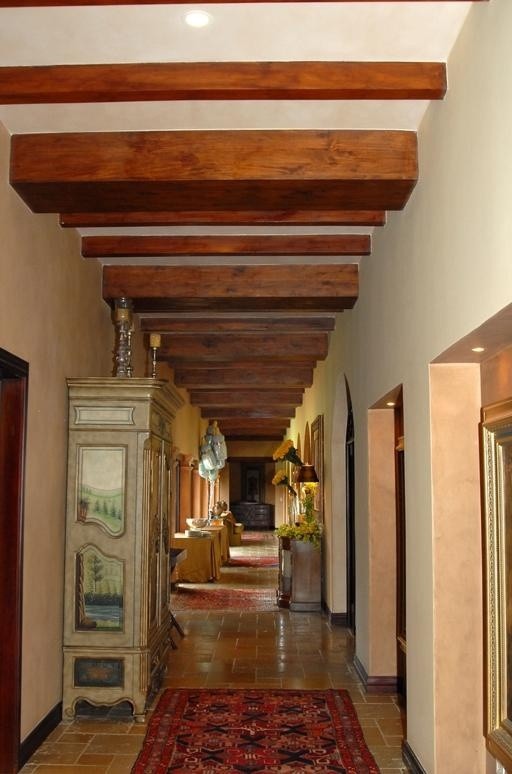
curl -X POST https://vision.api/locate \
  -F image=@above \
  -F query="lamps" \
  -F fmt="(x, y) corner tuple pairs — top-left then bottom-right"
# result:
(296, 462), (319, 484)
(112, 293), (135, 377)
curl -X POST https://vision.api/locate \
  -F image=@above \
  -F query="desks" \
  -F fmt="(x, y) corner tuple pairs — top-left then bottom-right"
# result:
(171, 524), (229, 582)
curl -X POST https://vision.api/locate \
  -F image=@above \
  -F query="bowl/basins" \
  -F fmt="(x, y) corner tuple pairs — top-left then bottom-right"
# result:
(185, 518), (208, 528)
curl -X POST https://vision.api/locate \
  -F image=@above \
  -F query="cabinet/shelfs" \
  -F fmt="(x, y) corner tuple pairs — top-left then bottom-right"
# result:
(231, 503), (273, 529)
(275, 534), (322, 612)
(64, 374), (186, 725)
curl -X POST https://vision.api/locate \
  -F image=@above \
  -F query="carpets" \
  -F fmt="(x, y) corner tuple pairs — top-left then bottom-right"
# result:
(170, 587), (280, 613)
(224, 555), (279, 567)
(241, 532), (278, 545)
(132, 685), (377, 774)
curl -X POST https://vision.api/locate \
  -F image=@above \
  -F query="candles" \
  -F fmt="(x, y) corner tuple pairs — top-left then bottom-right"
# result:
(149, 333), (161, 349)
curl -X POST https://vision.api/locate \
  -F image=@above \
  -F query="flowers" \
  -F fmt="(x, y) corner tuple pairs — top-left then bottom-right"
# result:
(271, 469), (297, 496)
(272, 438), (302, 466)
